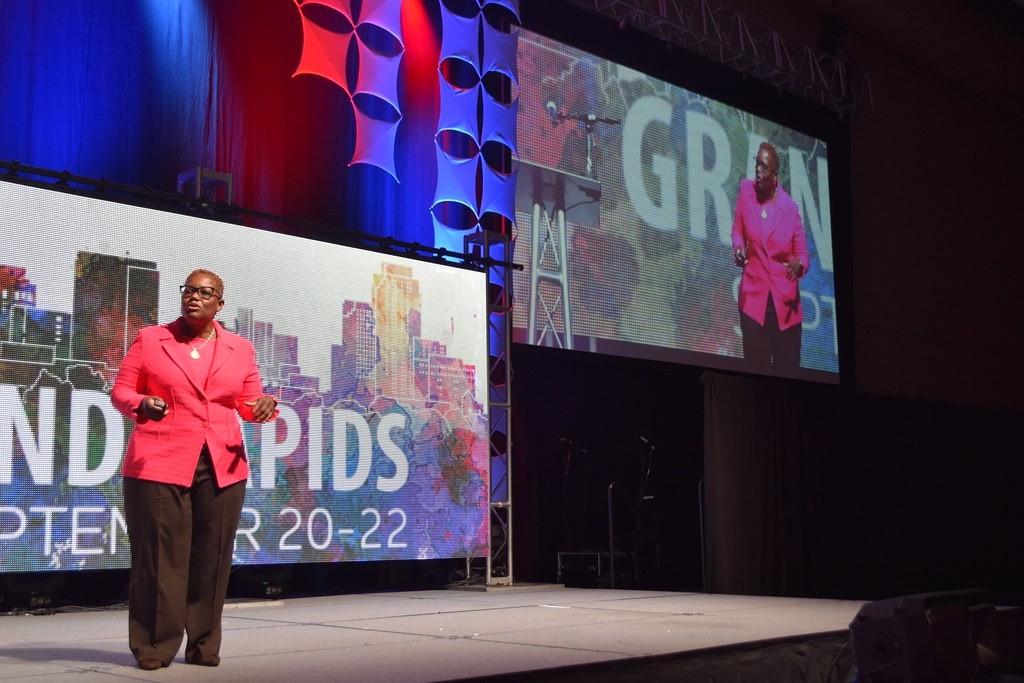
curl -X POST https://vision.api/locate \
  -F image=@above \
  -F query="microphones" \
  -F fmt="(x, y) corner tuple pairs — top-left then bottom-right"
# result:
(546, 101), (558, 126)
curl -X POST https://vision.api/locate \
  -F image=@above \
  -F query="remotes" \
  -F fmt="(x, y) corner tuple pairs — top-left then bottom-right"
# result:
(154, 400), (166, 412)
(738, 252), (746, 261)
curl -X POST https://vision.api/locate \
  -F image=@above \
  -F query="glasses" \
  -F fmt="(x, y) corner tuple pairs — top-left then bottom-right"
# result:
(180, 284), (222, 300)
(752, 157), (769, 173)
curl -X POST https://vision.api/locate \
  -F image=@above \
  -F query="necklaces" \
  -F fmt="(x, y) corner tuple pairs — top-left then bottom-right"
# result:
(185, 328), (214, 359)
(752, 187), (779, 219)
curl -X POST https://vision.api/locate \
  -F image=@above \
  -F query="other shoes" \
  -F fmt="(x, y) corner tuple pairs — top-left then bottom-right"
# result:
(200, 658), (219, 666)
(139, 659), (163, 670)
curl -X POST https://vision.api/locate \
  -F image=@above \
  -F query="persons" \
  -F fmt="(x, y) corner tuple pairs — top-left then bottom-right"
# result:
(733, 141), (811, 364)
(111, 269), (279, 670)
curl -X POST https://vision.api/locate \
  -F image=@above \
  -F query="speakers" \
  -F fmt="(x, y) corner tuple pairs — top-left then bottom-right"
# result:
(848, 590), (983, 683)
